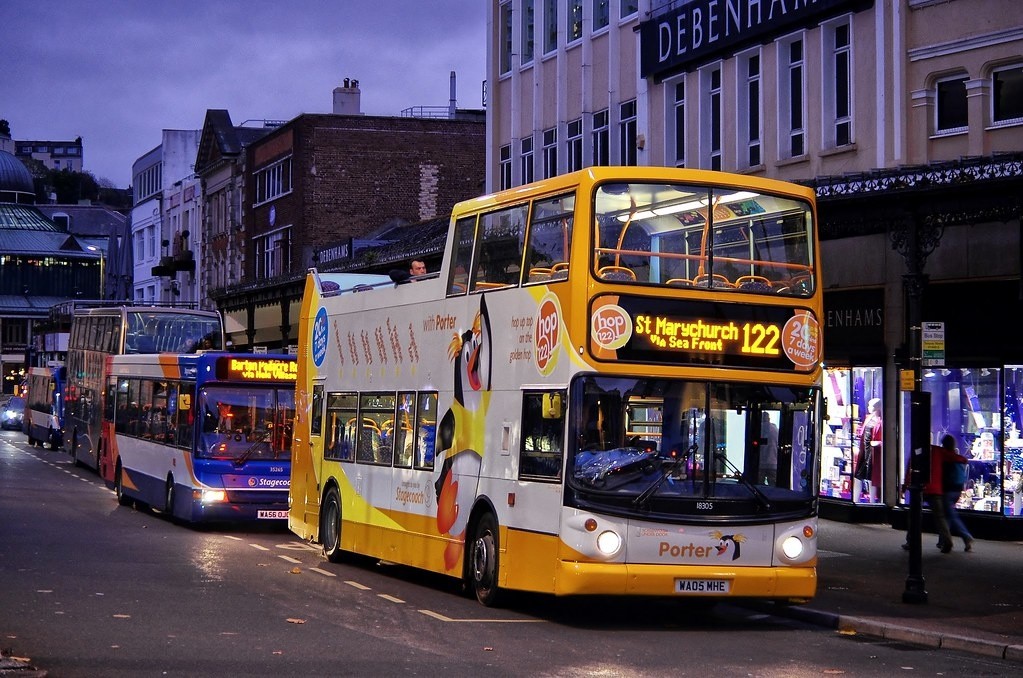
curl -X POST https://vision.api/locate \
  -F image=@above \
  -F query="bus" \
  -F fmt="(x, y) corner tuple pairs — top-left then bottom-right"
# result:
(287, 165), (829, 608)
(105, 350), (300, 525)
(20, 364), (67, 453)
(66, 307), (226, 474)
(46, 298), (134, 367)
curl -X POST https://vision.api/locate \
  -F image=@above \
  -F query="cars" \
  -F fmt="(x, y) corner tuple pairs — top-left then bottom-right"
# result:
(0, 396), (25, 430)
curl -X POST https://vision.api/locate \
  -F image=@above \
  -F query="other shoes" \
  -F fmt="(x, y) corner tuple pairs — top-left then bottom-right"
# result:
(941, 539), (952, 553)
(902, 543), (910, 549)
(964, 536), (974, 551)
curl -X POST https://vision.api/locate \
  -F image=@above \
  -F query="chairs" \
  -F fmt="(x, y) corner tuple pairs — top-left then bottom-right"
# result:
(71, 326), (206, 354)
(311, 415), (435, 468)
(322, 262), (814, 300)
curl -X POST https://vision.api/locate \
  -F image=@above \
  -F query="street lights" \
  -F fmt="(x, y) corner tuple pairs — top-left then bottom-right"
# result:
(87, 244), (104, 308)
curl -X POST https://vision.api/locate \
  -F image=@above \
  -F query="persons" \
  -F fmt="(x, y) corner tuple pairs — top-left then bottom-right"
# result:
(852, 398), (883, 503)
(176, 335), (194, 352)
(760, 411), (777, 487)
(202, 339), (214, 350)
(901, 445), (970, 554)
(398, 258), (429, 284)
(937, 436), (975, 551)
